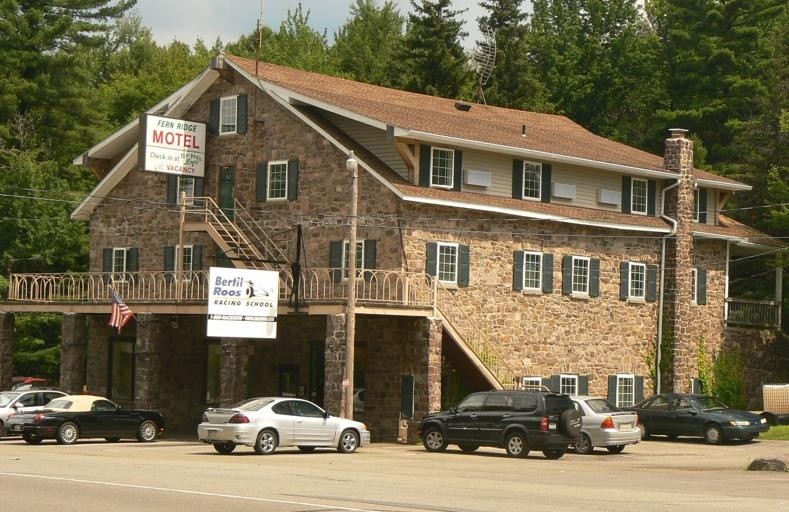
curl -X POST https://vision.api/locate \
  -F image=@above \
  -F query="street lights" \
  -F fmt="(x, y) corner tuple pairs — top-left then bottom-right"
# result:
(337, 149), (363, 421)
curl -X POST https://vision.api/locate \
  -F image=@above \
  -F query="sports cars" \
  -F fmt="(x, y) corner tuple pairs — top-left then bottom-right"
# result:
(4, 392), (167, 446)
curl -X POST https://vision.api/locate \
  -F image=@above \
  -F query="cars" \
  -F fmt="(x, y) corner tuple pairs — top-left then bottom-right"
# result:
(196, 395), (371, 456)
(563, 392), (643, 457)
(0, 377), (71, 438)
(619, 392), (769, 443)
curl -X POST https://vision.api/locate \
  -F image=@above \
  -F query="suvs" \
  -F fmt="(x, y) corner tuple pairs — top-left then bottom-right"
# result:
(414, 387), (580, 462)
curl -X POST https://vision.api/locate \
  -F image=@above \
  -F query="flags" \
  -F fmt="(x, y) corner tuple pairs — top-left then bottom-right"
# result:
(108, 289), (133, 334)
